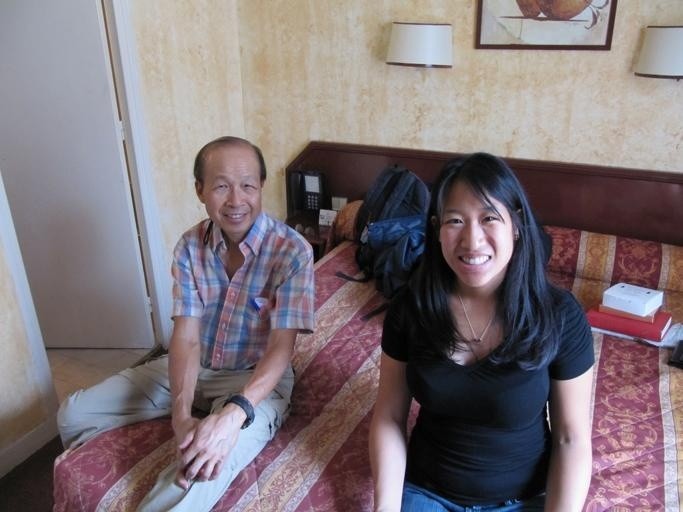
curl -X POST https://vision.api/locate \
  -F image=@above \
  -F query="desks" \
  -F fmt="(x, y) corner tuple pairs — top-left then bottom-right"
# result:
(283, 217), (334, 258)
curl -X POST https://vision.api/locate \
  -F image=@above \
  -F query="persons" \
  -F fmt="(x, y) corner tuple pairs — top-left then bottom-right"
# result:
(56, 134), (315, 511)
(365, 149), (595, 512)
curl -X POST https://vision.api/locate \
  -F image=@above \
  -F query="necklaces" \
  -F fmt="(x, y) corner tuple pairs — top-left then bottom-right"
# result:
(451, 281), (497, 348)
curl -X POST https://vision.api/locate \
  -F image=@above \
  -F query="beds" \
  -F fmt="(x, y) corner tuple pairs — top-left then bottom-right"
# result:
(51, 140), (682, 510)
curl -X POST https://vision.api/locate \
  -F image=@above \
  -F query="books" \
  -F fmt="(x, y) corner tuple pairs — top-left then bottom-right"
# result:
(597, 302), (661, 324)
(585, 300), (672, 342)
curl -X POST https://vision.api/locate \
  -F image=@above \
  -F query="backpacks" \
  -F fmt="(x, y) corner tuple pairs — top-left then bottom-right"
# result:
(352, 165), (433, 274)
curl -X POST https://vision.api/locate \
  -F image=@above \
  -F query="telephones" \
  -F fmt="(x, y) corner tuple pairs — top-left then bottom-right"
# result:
(290, 171), (326, 216)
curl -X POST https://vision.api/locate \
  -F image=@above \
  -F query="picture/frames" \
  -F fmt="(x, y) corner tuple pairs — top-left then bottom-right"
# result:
(473, 0), (617, 53)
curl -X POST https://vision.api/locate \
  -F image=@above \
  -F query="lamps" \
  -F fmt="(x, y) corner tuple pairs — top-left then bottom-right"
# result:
(633, 25), (683, 82)
(385, 21), (454, 69)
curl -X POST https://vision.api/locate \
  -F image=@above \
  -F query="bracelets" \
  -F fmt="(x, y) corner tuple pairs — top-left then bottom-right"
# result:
(221, 394), (254, 430)
